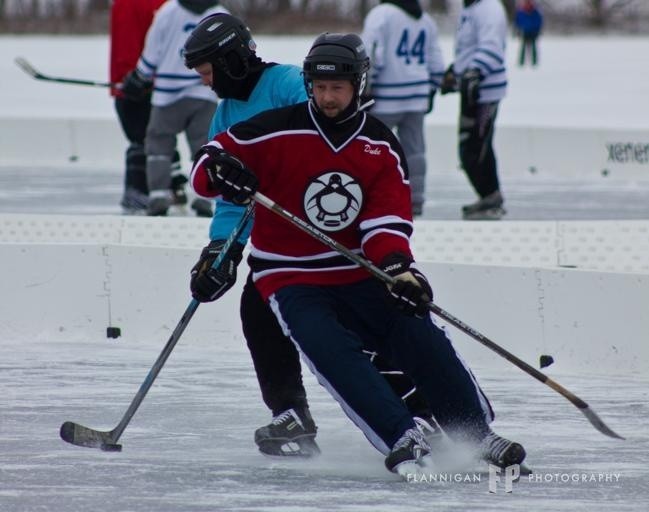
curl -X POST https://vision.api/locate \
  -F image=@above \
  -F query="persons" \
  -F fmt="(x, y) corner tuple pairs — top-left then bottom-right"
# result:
(357, 1), (444, 216)
(181, 14), (446, 458)
(115, 1), (232, 219)
(515, 1), (542, 65)
(189, 30), (525, 471)
(442, 0), (506, 218)
(108, 2), (187, 209)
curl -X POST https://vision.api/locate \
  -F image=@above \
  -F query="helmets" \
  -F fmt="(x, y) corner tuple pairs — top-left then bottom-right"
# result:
(182, 12), (256, 79)
(303, 32), (370, 97)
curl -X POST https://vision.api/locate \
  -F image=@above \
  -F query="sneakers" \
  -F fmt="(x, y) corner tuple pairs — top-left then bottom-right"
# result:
(254, 406), (317, 444)
(462, 191), (503, 215)
(385, 428), (432, 472)
(411, 416), (444, 445)
(171, 183), (187, 205)
(147, 198), (169, 215)
(121, 189), (150, 209)
(475, 432), (526, 467)
(192, 198), (213, 217)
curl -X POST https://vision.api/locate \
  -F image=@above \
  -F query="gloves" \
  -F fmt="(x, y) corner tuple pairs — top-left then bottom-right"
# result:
(442, 70), (456, 95)
(380, 251), (433, 318)
(190, 238), (245, 303)
(462, 69), (481, 103)
(118, 70), (151, 103)
(205, 149), (258, 206)
